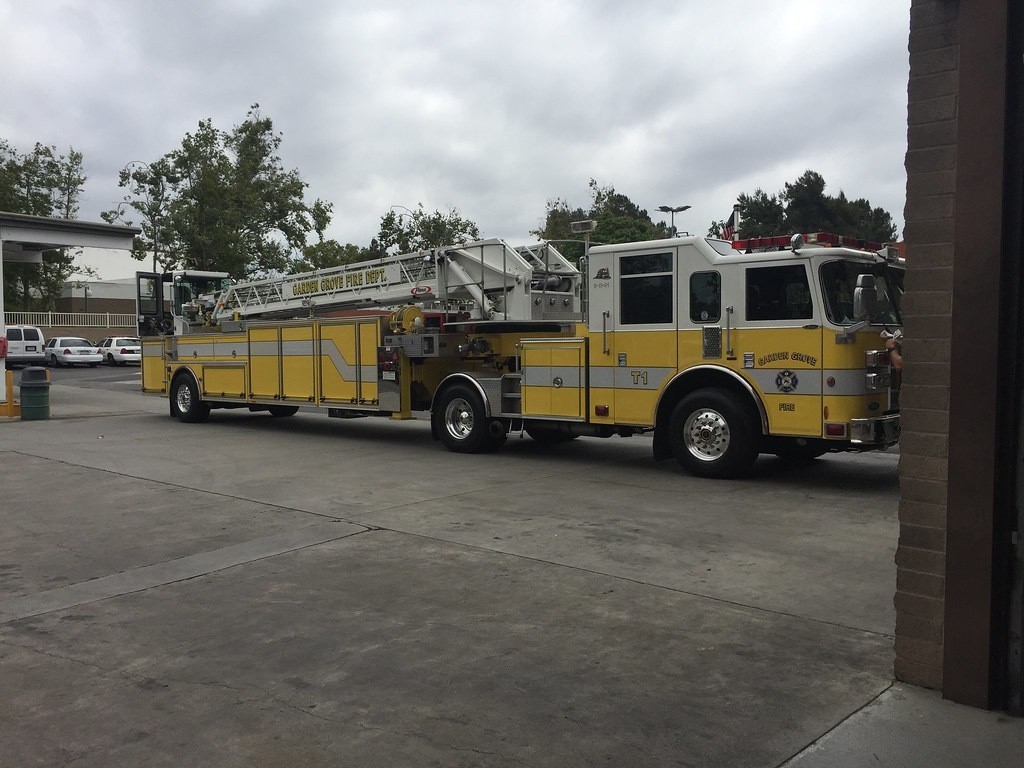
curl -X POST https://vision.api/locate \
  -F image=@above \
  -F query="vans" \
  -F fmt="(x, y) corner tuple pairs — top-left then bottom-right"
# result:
(3, 325), (46, 369)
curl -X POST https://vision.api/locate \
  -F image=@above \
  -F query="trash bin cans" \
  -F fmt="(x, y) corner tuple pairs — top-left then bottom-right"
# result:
(17, 366), (52, 420)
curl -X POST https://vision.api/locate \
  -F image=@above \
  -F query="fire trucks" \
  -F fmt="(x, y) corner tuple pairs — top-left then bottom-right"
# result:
(134, 205), (904, 479)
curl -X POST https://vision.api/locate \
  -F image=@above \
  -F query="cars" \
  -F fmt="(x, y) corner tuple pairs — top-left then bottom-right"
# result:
(93, 337), (142, 366)
(45, 337), (104, 368)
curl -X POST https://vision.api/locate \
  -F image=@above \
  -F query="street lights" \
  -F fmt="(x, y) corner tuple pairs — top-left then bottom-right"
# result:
(658, 205), (690, 236)
(85, 285), (91, 313)
